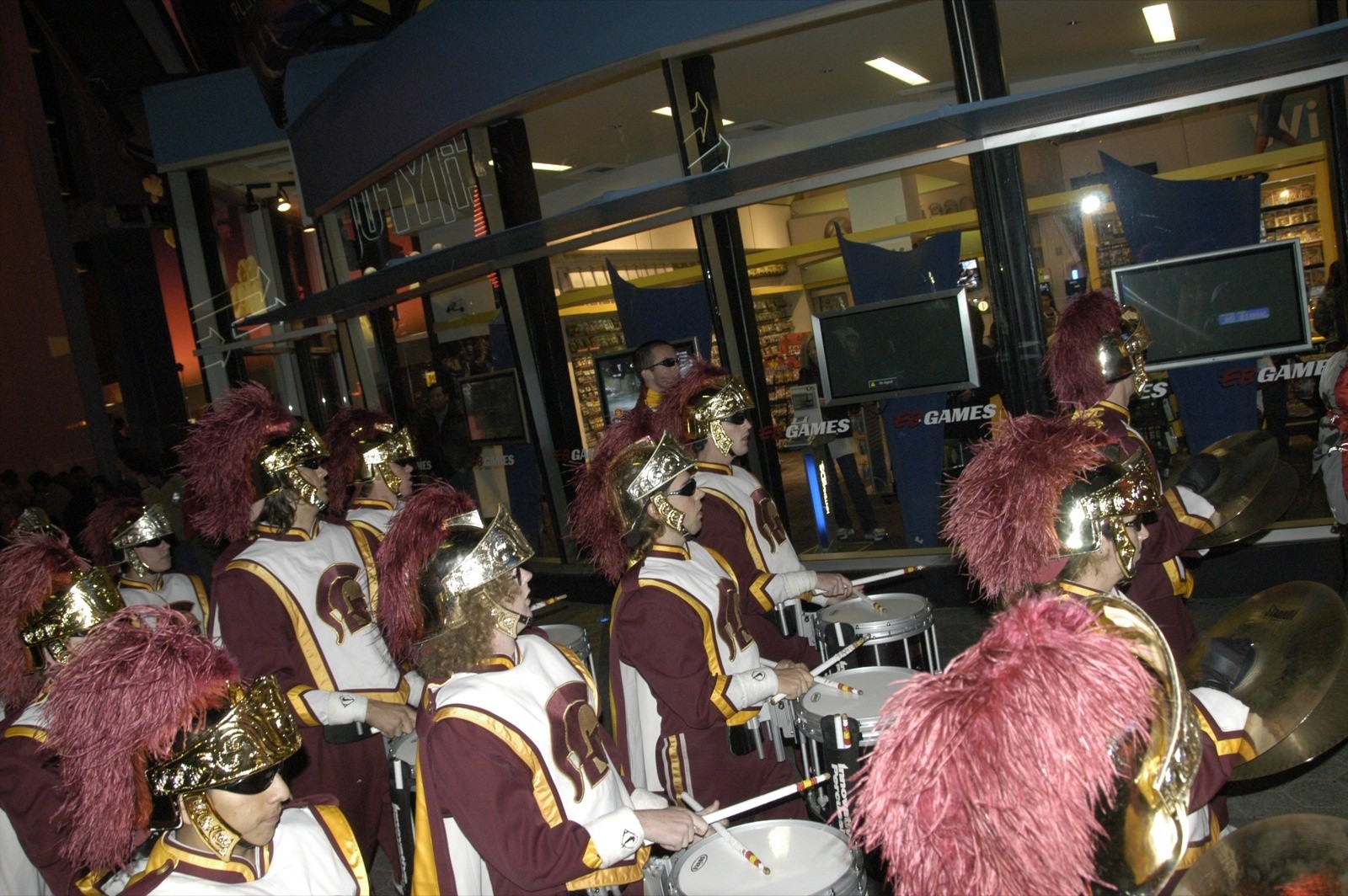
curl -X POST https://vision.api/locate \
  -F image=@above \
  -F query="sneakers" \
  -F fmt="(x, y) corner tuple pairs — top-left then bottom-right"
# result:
(836, 527), (855, 541)
(864, 527), (888, 542)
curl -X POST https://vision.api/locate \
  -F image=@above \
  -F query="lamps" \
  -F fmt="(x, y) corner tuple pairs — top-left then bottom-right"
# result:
(276, 180), (297, 212)
(243, 183), (273, 214)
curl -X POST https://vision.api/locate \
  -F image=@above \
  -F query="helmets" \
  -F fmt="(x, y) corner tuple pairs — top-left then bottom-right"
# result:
(0, 382), (533, 865)
(567, 408), (697, 583)
(828, 579), (1201, 896)
(938, 407), (1161, 604)
(1040, 292), (1153, 409)
(655, 360), (755, 458)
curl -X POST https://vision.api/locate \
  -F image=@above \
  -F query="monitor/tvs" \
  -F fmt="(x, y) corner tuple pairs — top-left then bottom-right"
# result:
(811, 287), (980, 406)
(1111, 236), (1312, 372)
(457, 368), (531, 446)
(593, 337), (701, 430)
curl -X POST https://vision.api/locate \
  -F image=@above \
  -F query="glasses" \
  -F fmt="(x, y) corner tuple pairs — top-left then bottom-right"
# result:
(509, 566), (522, 586)
(1122, 513), (1145, 530)
(720, 411), (749, 424)
(390, 459), (408, 466)
(666, 478), (697, 496)
(214, 756), (297, 794)
(133, 535), (162, 546)
(638, 358), (680, 371)
(296, 460), (321, 469)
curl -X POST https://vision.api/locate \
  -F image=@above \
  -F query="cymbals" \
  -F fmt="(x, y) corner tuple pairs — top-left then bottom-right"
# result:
(1158, 427), (1302, 550)
(1187, 577), (1348, 782)
(1170, 811), (1348, 896)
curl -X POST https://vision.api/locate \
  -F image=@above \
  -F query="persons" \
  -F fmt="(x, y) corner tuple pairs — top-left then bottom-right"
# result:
(633, 340), (680, 433)
(858, 602), (1157, 896)
(1313, 261), (1341, 339)
(51, 618), (397, 896)
(323, 410), (419, 533)
(424, 384), (482, 514)
(574, 421), (814, 826)
(181, 388), (426, 896)
(83, 503), (208, 634)
(378, 487), (729, 896)
(1, 528), (130, 895)
(1317, 348), (1348, 538)
(656, 368), (864, 616)
(0, 420), (188, 556)
(1042, 293), (1059, 326)
(947, 419), (1250, 896)
(947, 305), (1004, 449)
(1048, 290), (1213, 665)
(798, 334), (825, 399)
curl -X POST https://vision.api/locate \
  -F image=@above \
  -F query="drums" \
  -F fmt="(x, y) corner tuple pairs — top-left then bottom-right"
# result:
(387, 723), (420, 845)
(792, 666), (952, 842)
(816, 590), (939, 676)
(536, 623), (597, 687)
(668, 816), (869, 896)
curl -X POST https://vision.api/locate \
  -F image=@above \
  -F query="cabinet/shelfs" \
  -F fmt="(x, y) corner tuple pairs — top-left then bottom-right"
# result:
(1102, 198), (1321, 334)
